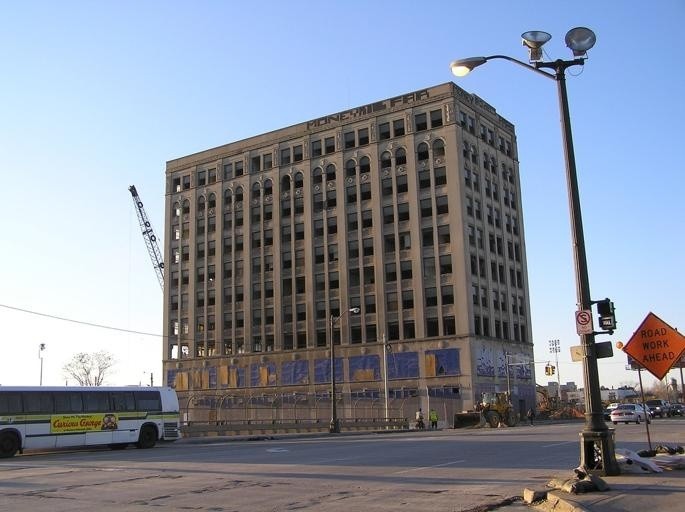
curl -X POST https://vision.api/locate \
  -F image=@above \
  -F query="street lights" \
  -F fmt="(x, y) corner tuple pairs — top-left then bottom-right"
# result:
(330, 306), (358, 432)
(548, 339), (561, 398)
(450, 28), (615, 475)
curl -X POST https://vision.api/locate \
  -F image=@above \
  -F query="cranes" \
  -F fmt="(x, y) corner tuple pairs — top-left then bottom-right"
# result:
(127, 183), (165, 294)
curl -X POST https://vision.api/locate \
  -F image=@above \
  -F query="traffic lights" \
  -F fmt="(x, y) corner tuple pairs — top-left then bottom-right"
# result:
(544, 365), (555, 375)
(598, 301), (614, 328)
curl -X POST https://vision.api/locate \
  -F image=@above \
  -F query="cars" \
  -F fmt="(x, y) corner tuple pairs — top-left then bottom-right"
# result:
(606, 399), (685, 424)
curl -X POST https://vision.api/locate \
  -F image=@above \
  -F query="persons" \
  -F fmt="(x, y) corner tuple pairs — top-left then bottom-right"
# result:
(415, 408), (424, 429)
(527, 407), (536, 427)
(430, 408), (438, 429)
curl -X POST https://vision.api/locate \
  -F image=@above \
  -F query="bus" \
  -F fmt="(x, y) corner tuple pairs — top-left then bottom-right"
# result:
(1, 386), (182, 457)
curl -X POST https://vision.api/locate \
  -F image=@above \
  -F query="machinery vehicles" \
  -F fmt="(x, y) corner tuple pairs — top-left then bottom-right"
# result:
(452, 390), (515, 429)
(536, 384), (584, 419)
